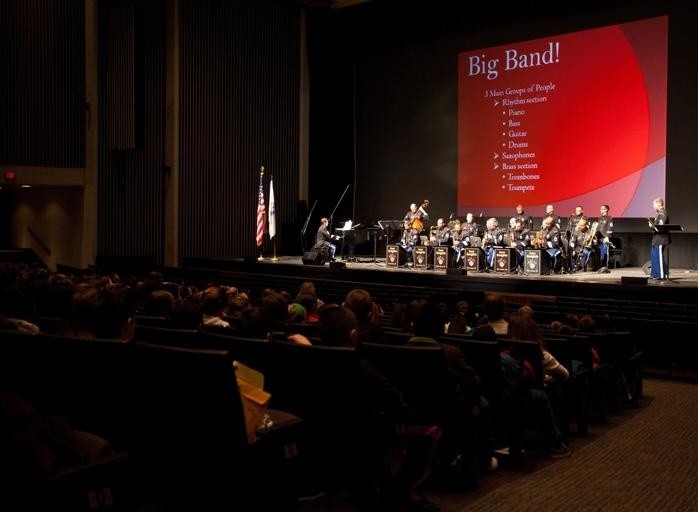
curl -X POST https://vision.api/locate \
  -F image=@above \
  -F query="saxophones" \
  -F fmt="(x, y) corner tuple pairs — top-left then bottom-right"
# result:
(531, 227), (546, 245)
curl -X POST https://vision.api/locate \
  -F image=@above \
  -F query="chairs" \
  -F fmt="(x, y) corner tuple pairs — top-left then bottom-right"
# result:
(608, 236), (623, 268)
(1, 278), (697, 511)
(314, 235), (330, 258)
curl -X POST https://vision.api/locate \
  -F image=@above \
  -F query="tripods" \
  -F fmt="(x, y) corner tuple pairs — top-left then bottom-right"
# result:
(655, 230), (680, 284)
(367, 232), (384, 269)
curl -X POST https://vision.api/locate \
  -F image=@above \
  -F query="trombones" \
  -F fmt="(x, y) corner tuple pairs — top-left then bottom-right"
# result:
(576, 222), (598, 265)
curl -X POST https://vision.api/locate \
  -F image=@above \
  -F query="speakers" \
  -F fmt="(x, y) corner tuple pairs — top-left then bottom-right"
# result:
(302, 251), (326, 265)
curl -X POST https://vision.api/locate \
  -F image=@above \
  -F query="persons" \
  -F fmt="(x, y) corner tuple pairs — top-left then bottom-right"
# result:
(1, 260), (647, 511)
(398, 203), (614, 274)
(317, 217), (337, 263)
(647, 197), (672, 279)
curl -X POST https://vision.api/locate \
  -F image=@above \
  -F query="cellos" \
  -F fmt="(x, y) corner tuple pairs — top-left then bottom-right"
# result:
(403, 200), (430, 233)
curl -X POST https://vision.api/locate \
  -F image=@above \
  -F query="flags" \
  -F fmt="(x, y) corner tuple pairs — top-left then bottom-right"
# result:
(267, 179), (277, 241)
(256, 177), (268, 248)
